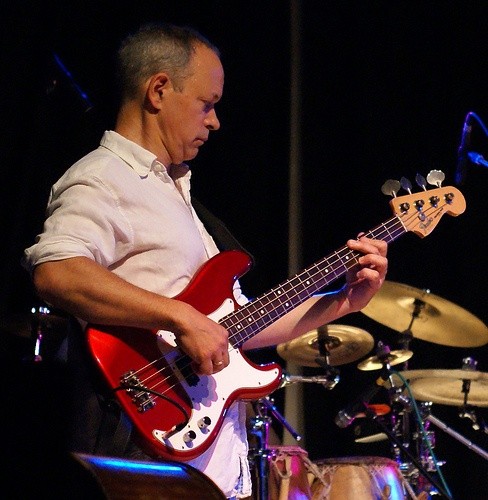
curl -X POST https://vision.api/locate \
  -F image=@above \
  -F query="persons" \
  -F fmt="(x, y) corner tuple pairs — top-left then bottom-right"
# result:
(25, 19), (388, 500)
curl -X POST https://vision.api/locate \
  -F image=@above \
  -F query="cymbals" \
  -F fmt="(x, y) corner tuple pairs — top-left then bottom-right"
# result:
(357, 350), (413, 371)
(276, 324), (375, 367)
(375, 369), (488, 407)
(360, 280), (488, 347)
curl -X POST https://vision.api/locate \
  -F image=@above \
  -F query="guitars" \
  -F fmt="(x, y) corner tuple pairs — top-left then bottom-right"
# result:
(83, 170), (466, 461)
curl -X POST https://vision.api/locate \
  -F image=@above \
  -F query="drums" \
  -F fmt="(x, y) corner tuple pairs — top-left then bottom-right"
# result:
(248, 445), (313, 500)
(307, 456), (405, 500)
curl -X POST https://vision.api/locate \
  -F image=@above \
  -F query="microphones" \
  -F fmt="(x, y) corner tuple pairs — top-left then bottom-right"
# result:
(334, 370), (391, 427)
(54, 56), (94, 112)
(455, 113), (473, 182)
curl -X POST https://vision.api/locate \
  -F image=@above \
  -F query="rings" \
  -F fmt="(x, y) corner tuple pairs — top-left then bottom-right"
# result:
(213, 360), (224, 365)
